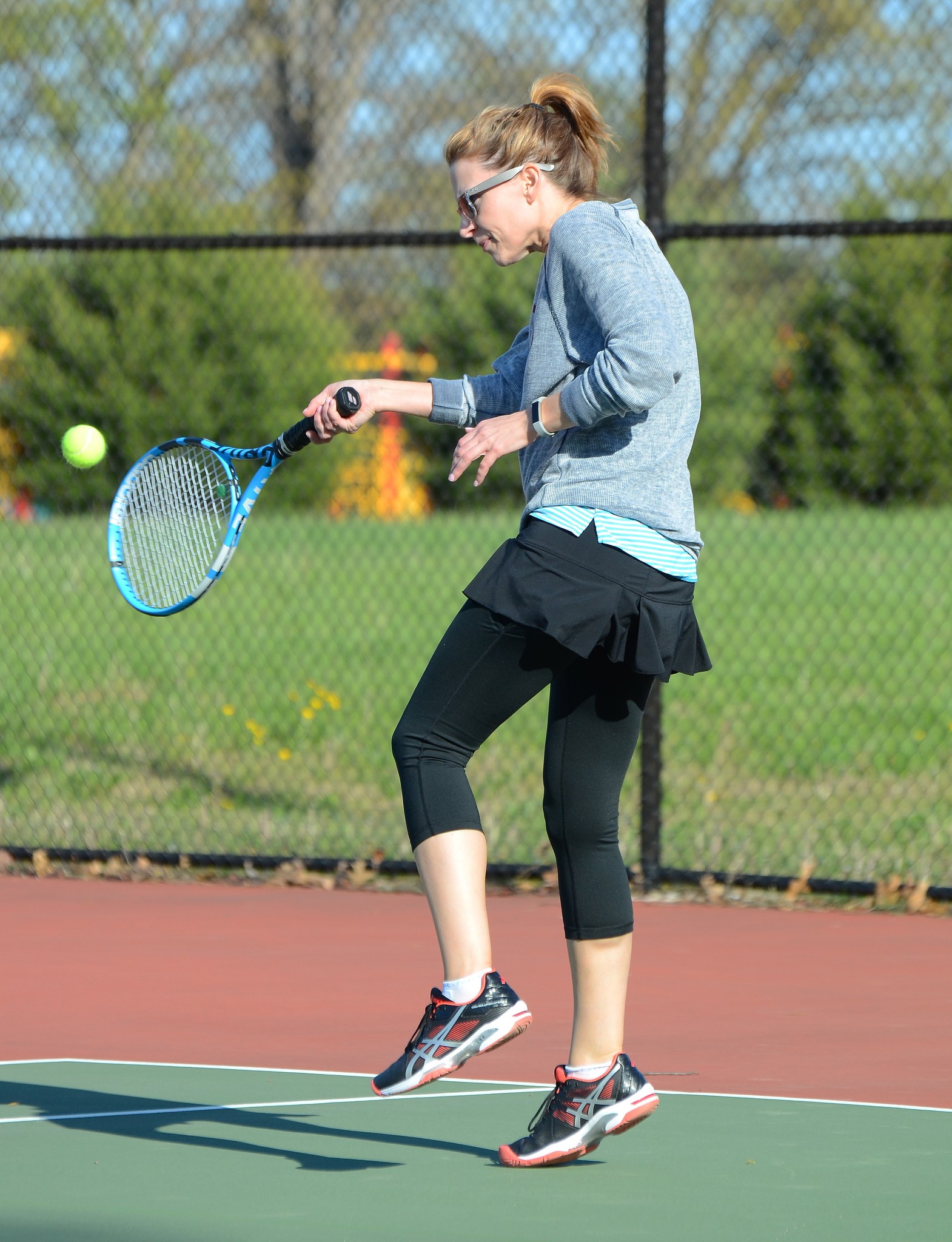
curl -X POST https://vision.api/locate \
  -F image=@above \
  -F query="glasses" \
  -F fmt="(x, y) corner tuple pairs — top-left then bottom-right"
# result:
(456, 163), (555, 221)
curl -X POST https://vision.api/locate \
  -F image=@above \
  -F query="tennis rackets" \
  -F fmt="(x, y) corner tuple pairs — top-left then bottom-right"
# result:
(106, 386), (363, 618)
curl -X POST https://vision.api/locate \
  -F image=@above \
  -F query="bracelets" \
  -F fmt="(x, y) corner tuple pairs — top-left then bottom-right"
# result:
(532, 396), (559, 438)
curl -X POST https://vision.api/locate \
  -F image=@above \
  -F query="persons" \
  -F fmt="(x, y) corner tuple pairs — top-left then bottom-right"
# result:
(292, 77), (719, 1170)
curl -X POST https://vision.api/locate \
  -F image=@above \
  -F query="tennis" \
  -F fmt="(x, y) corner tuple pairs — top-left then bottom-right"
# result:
(60, 424), (108, 470)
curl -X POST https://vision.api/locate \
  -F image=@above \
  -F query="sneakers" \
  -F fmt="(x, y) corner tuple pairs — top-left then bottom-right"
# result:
(498, 1051), (660, 1167)
(371, 970), (532, 1098)
